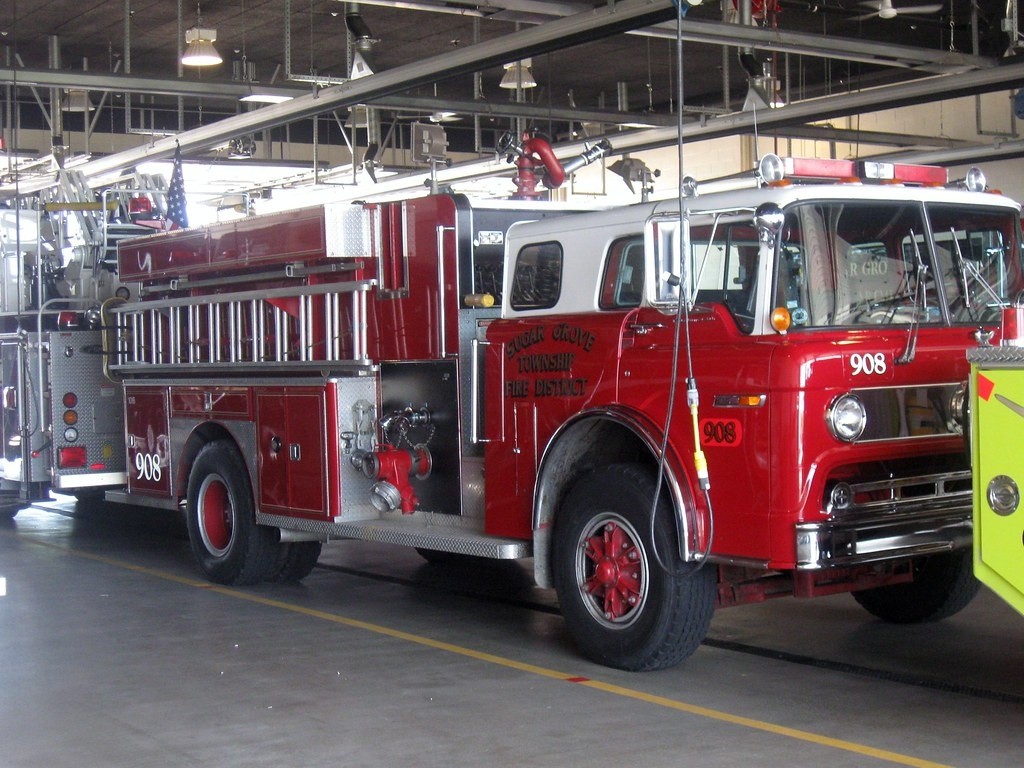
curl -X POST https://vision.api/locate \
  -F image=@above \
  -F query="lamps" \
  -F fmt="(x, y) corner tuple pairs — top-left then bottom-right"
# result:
(500, 56), (536, 89)
(180, 39), (224, 67)
(345, 106), (367, 128)
(762, 62), (784, 108)
(62, 89), (95, 112)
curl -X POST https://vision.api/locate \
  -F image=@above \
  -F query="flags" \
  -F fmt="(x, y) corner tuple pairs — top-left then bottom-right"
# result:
(166, 139), (189, 228)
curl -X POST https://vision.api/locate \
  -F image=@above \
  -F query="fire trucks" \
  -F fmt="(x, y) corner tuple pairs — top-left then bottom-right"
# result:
(0, 124), (1024, 673)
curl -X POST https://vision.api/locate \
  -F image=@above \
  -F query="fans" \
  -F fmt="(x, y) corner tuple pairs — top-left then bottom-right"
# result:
(845, 0), (943, 22)
